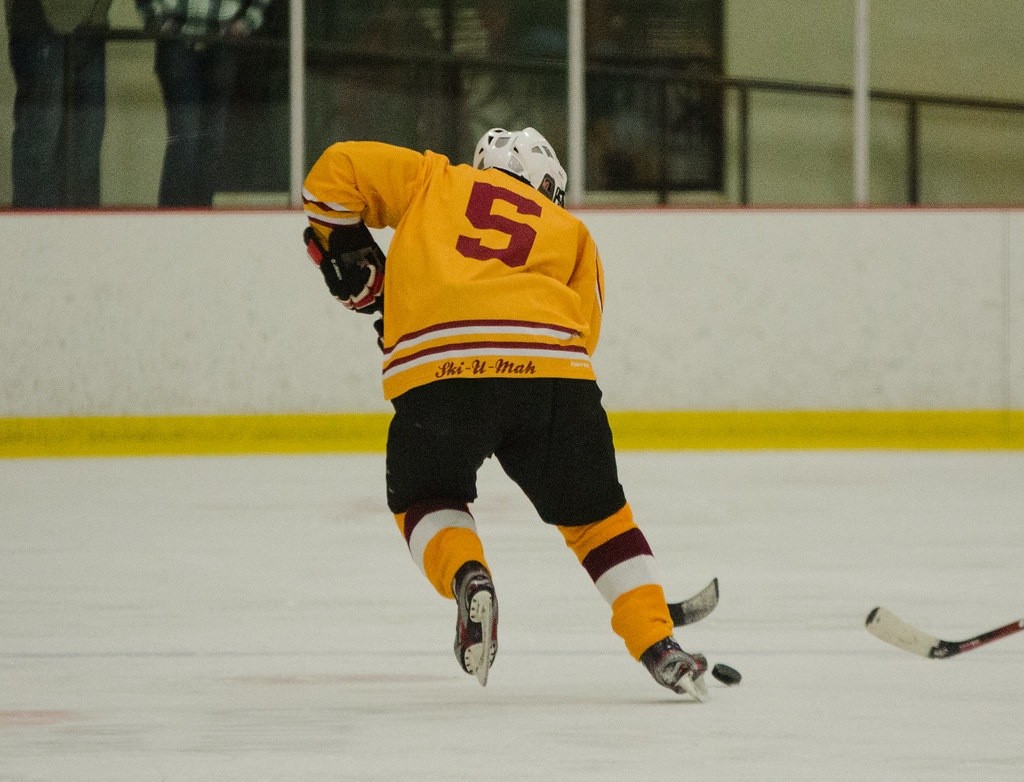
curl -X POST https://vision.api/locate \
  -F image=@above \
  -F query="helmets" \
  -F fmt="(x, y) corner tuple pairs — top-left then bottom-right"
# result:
(473, 126), (568, 206)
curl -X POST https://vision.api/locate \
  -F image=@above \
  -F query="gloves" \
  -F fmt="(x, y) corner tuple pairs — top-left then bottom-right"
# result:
(303, 223), (386, 315)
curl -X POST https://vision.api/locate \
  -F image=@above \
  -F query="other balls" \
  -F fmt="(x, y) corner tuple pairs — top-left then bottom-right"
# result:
(712, 663), (742, 685)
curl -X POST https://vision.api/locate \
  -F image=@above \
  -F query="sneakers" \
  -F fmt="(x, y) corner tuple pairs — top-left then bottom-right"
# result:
(453, 560), (499, 688)
(641, 636), (711, 702)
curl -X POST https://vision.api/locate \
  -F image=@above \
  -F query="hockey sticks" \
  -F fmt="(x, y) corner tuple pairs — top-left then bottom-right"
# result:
(340, 255), (718, 627)
(865, 606), (1023, 660)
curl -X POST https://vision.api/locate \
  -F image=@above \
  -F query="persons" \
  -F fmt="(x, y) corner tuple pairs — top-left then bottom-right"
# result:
(302, 124), (708, 701)
